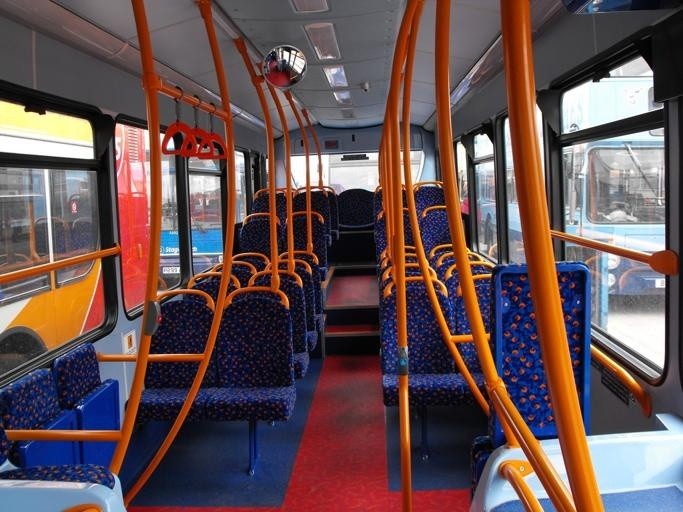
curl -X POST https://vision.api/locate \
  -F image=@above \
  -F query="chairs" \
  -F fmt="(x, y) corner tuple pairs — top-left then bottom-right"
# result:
(375, 184), (495, 406)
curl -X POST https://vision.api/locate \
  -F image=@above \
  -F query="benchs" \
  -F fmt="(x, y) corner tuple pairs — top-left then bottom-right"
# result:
(334, 188), (375, 232)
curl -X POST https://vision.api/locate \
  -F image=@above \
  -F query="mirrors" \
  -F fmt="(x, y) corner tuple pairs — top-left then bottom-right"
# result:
(261, 43), (306, 91)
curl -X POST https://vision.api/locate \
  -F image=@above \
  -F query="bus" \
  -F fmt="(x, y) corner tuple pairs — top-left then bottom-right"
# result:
(145, 141), (257, 290)
(1, 99), (152, 381)
(453, 118), (666, 300)
